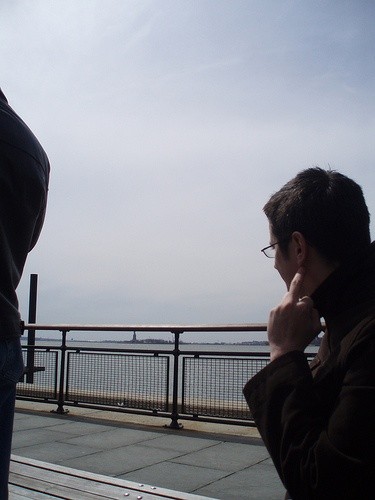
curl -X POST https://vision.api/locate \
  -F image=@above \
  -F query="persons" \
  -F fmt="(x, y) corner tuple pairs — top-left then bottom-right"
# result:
(242, 162), (375, 500)
(0, 89), (51, 500)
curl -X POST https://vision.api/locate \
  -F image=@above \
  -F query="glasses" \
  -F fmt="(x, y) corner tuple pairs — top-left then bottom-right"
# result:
(261, 239), (291, 258)
